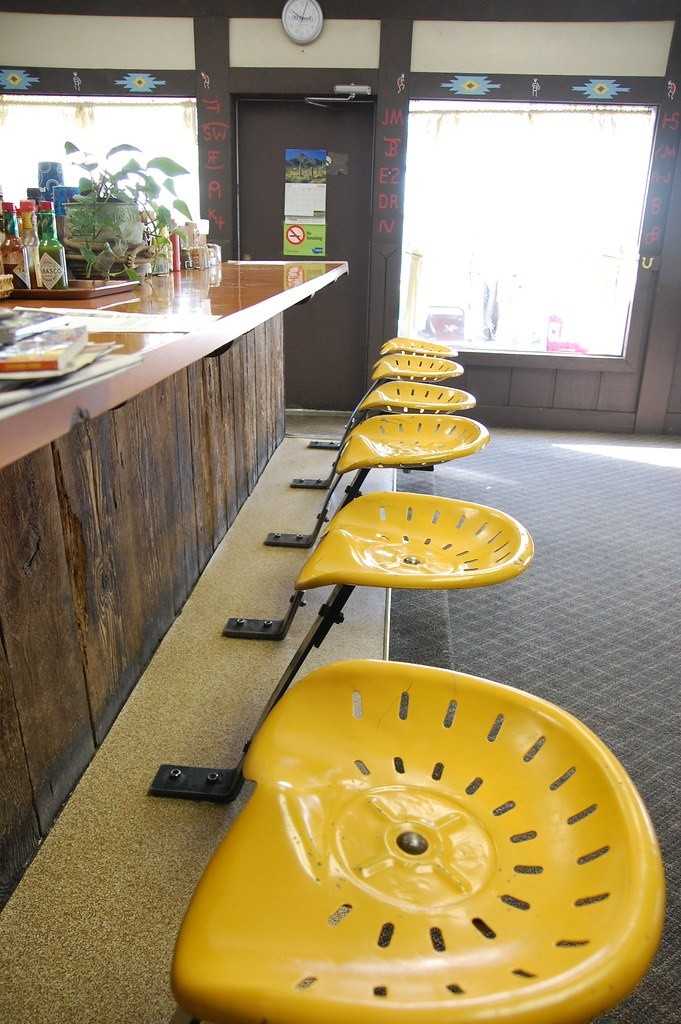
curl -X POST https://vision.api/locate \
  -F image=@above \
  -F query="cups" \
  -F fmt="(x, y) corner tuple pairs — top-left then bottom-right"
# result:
(53, 185), (79, 216)
(38, 161), (64, 201)
(179, 226), (221, 270)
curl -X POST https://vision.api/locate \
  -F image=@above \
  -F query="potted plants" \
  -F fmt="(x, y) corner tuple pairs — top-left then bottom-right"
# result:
(62, 139), (194, 287)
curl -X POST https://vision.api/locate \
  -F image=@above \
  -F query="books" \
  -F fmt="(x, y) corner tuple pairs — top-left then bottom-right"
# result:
(0, 308), (143, 407)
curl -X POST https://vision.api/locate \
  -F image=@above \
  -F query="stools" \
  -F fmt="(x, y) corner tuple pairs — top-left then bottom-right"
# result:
(141, 337), (669, 1024)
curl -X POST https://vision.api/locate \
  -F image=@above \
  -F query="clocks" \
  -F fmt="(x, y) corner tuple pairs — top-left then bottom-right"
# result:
(281, 0), (324, 46)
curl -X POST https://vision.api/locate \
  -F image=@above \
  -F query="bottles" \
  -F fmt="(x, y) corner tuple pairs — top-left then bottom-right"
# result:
(0, 202), (31, 289)
(20, 200), (43, 289)
(35, 200), (68, 290)
